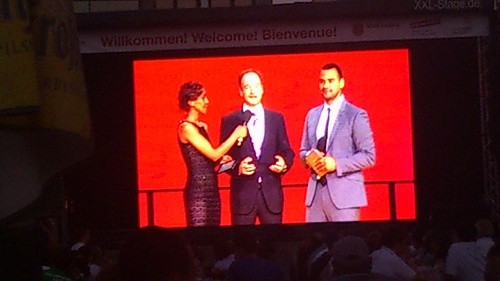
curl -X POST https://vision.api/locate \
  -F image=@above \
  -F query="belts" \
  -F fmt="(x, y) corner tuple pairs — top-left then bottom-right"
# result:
(258, 182), (262, 189)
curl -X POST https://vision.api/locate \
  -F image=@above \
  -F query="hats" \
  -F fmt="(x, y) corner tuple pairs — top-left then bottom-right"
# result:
(334, 236), (369, 264)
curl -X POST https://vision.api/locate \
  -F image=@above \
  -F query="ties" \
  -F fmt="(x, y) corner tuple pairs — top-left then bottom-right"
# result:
(324, 108), (330, 139)
(252, 116), (258, 126)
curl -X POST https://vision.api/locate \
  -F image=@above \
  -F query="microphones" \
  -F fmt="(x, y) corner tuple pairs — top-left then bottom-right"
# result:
(316, 137), (326, 179)
(237, 119), (247, 146)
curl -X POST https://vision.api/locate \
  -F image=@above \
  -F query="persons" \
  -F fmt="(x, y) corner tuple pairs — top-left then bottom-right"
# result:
(298, 62), (376, 222)
(218, 70), (296, 225)
(176, 80), (247, 226)
(0, 199), (500, 281)
